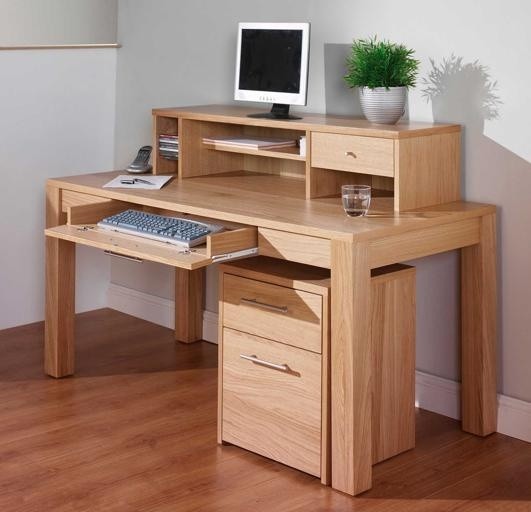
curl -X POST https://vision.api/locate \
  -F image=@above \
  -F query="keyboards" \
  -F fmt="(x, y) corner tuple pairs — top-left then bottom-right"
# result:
(96, 208), (225, 248)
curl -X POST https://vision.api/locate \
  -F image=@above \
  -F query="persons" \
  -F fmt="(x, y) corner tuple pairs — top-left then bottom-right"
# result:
(159, 133), (179, 160)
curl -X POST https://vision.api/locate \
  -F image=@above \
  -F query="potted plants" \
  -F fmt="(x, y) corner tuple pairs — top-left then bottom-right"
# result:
(343, 35), (420, 127)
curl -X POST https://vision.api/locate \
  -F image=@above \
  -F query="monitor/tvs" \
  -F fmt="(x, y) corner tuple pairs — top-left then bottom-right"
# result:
(234, 20), (310, 120)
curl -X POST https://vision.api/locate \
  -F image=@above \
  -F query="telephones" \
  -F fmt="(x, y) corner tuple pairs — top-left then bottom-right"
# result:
(125, 146), (152, 173)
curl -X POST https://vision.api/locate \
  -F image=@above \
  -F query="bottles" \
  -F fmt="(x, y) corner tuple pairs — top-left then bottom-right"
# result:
(299, 135), (306, 158)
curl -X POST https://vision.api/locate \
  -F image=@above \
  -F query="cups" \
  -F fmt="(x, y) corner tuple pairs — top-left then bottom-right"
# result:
(340, 185), (372, 219)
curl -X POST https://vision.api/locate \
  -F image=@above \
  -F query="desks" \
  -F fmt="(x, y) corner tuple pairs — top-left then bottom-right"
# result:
(43, 106), (499, 497)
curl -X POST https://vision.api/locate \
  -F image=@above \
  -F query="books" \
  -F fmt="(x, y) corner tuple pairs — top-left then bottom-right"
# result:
(102, 173), (173, 190)
(203, 141), (282, 151)
(202, 134), (296, 147)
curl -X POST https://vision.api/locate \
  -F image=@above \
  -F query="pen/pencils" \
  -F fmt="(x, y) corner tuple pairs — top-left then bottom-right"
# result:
(134, 178), (152, 185)
